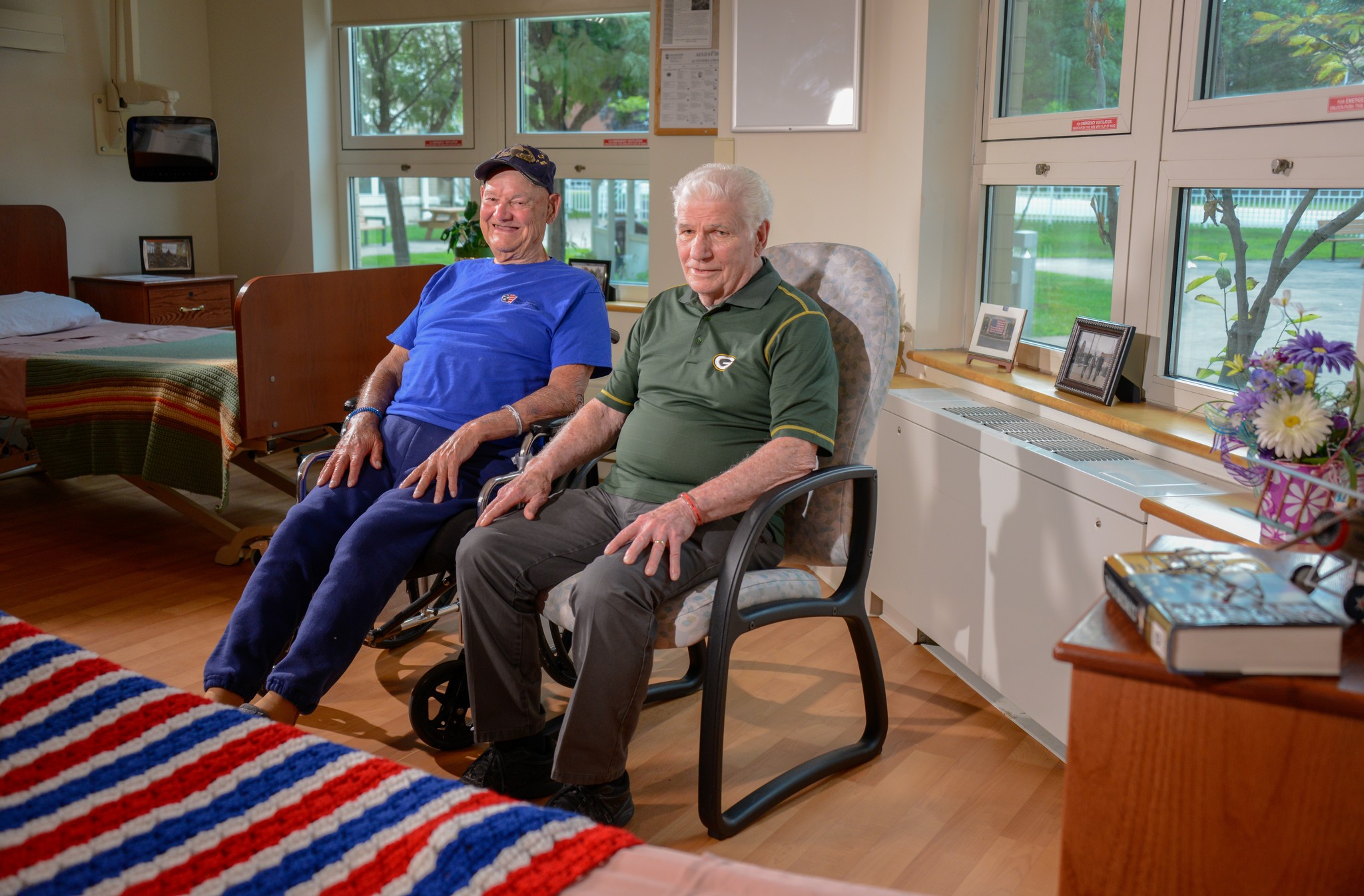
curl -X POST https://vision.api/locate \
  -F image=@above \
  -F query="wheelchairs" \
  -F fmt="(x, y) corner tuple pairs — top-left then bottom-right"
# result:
(296, 328), (621, 752)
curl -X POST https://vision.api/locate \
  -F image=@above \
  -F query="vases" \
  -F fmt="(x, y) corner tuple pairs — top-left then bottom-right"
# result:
(1250, 449), (1354, 549)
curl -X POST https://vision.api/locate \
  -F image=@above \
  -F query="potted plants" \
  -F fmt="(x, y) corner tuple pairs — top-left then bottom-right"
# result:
(441, 201), (494, 259)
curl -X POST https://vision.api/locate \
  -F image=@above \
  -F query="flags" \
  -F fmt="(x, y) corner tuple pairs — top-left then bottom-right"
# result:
(989, 319), (1006, 335)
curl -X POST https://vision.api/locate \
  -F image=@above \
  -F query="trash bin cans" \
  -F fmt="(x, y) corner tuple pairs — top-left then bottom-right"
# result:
(605, 218), (648, 279)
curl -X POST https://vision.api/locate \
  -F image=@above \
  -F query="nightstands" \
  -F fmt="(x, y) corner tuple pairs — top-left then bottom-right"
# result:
(71, 273), (238, 330)
(1054, 569), (1364, 896)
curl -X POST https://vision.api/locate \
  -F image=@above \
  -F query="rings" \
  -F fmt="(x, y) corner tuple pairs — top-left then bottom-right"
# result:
(652, 540), (666, 546)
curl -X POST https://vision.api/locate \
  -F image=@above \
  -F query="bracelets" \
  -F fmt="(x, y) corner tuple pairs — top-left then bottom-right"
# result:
(677, 492), (704, 526)
(499, 404), (523, 438)
(340, 406), (383, 439)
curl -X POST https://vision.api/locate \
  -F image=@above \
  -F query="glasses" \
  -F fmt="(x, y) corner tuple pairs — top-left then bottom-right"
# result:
(1158, 548), (1265, 610)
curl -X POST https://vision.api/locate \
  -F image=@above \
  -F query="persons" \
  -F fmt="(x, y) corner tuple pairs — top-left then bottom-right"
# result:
(1080, 350), (1104, 382)
(456, 165), (839, 828)
(202, 145), (612, 726)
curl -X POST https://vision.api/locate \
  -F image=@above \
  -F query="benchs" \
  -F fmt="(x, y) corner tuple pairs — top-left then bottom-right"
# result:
(1318, 219), (1364, 263)
(409, 217), (465, 228)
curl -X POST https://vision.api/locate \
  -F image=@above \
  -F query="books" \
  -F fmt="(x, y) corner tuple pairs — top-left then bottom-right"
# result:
(1103, 545), (1347, 679)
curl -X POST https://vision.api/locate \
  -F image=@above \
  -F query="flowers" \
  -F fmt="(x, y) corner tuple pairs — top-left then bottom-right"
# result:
(1208, 319), (1360, 473)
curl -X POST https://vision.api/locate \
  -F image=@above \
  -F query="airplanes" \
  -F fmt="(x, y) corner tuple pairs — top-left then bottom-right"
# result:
(1229, 457), (1364, 623)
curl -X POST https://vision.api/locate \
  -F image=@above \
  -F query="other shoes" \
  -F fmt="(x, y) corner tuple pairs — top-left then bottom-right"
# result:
(454, 733), (561, 802)
(542, 768), (635, 828)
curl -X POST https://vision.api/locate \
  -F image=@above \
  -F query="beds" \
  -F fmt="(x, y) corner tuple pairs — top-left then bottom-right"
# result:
(0, 613), (911, 896)
(0, 200), (449, 571)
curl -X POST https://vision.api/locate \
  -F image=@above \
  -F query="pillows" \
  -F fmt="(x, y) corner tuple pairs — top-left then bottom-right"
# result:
(0, 290), (100, 339)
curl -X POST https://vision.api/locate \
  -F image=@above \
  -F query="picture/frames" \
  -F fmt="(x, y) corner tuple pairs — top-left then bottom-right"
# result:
(966, 302), (1027, 374)
(1055, 315), (1147, 407)
(139, 235), (195, 274)
(568, 258), (616, 301)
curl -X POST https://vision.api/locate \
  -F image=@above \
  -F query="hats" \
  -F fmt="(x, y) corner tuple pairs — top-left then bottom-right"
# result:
(474, 143), (556, 195)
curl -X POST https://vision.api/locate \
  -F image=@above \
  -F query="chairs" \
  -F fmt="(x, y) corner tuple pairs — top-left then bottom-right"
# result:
(540, 237), (914, 835)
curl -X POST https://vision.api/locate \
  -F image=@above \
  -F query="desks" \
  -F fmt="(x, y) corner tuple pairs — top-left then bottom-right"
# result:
(422, 207), (466, 240)
